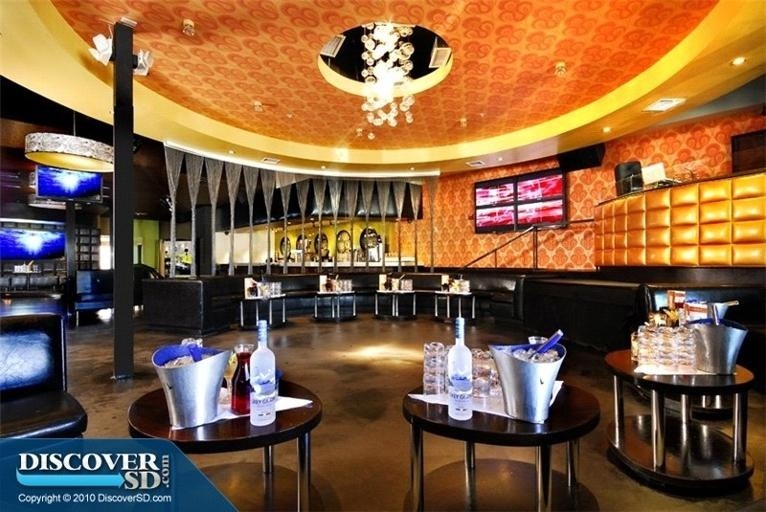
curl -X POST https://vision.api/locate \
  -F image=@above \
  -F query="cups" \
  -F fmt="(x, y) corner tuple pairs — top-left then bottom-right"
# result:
(528, 337), (547, 345)
(426, 403), (443, 418)
(422, 342), (505, 411)
(631, 326), (696, 369)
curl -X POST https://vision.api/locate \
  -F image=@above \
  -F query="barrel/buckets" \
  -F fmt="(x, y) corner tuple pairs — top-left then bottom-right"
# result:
(151, 344), (235, 428)
(486, 342), (568, 424)
(684, 317), (749, 375)
(683, 301), (729, 323)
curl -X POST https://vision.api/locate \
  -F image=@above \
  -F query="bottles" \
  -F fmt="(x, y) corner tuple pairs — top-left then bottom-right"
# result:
(447, 317), (472, 422)
(229, 344), (253, 416)
(250, 320), (275, 427)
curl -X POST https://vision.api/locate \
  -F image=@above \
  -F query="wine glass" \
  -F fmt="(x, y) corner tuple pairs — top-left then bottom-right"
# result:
(223, 353), (237, 403)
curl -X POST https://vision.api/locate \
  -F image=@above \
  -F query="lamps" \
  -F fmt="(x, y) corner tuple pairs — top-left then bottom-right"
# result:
(24, 112), (115, 173)
(360, 23), (416, 141)
(131, 50), (154, 79)
(89, 32), (114, 65)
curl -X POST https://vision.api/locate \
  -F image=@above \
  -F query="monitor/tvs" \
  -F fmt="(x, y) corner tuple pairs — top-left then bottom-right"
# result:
(516, 198), (567, 231)
(474, 179), (517, 207)
(516, 169), (566, 205)
(35, 164), (103, 204)
(475, 204), (517, 234)
(0, 311), (67, 403)
(0, 226), (68, 263)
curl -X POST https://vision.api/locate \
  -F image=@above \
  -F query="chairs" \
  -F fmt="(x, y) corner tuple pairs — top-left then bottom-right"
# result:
(1, 312), (89, 440)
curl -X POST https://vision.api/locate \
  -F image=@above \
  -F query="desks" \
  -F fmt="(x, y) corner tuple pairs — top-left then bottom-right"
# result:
(401, 380), (600, 511)
(606, 346), (757, 501)
(232, 289), (481, 331)
(129, 382), (325, 512)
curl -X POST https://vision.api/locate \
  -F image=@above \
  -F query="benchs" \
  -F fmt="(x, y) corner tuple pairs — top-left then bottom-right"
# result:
(141, 273), (517, 336)
(69, 269), (113, 329)
